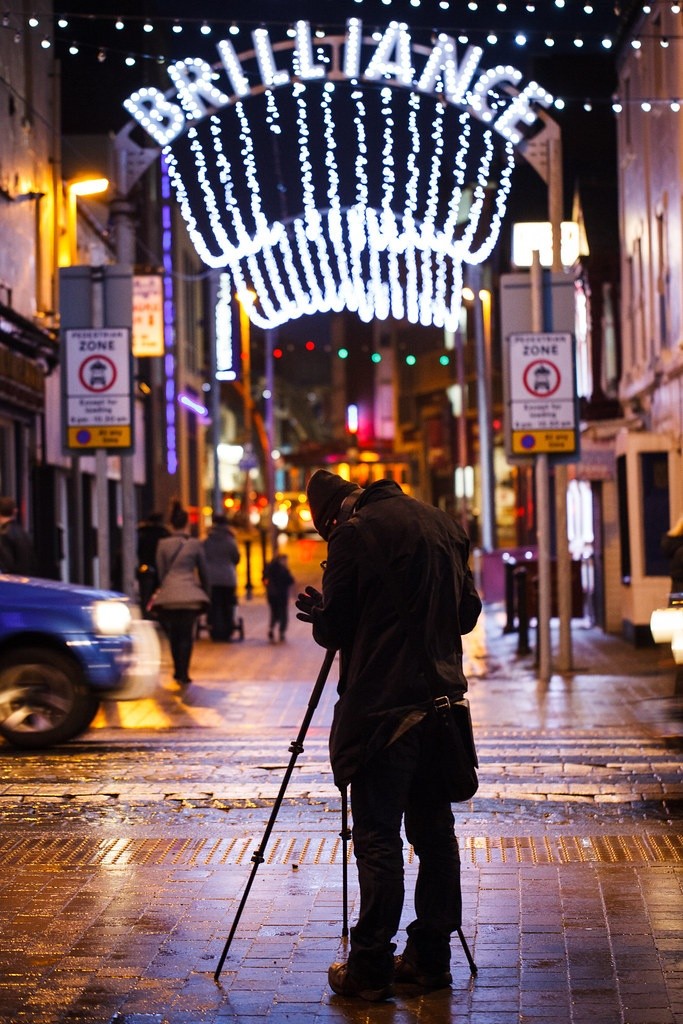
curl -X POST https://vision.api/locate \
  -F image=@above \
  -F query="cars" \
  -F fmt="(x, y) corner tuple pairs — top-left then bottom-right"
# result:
(223, 492), (320, 540)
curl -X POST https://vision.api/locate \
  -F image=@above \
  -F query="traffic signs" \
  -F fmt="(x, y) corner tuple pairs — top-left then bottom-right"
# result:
(64, 327), (130, 448)
(510, 333), (575, 456)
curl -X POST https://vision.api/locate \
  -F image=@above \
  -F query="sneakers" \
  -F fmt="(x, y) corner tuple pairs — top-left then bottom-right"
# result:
(330, 962), (398, 1003)
(389, 954), (453, 991)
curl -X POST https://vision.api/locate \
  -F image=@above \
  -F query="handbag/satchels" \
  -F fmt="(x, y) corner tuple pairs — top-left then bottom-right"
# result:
(437, 703), (479, 803)
(146, 589), (162, 618)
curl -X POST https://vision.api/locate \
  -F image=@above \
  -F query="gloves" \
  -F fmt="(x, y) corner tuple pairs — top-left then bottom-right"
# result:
(296, 586), (326, 624)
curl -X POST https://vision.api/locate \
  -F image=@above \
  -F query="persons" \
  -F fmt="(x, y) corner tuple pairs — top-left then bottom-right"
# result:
(295, 470), (482, 1003)
(135, 511), (172, 620)
(202, 511), (241, 642)
(154, 498), (211, 684)
(264, 553), (295, 642)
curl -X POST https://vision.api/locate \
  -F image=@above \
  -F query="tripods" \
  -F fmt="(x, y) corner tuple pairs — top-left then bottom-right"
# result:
(213, 645), (478, 981)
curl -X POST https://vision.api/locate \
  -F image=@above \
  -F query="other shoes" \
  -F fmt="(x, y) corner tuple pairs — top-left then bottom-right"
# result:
(173, 674), (191, 683)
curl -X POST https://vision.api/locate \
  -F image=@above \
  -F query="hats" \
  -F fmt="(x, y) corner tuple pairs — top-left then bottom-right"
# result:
(307, 470), (359, 540)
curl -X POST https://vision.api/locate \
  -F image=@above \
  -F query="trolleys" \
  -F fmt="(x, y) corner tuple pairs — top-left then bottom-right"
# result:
(192, 580), (244, 640)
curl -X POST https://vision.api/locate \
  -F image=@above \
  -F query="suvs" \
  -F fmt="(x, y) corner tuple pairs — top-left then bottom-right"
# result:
(0, 574), (138, 749)
